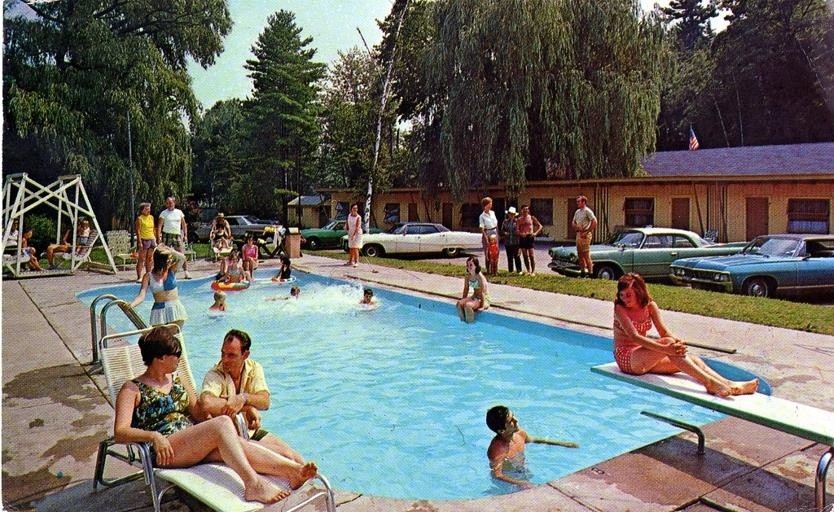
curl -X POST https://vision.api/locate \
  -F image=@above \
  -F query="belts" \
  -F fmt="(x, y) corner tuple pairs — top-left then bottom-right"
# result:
(484, 227), (497, 230)
(575, 229), (588, 232)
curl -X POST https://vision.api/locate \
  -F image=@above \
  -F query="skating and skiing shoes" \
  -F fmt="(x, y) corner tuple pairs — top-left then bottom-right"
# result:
(508, 271), (535, 277)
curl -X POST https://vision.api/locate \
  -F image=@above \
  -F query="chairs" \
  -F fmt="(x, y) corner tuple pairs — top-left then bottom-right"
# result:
(88, 321), (336, 512)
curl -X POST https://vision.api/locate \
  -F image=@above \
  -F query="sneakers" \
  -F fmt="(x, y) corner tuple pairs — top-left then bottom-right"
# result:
(184, 271), (192, 279)
(136, 275), (142, 284)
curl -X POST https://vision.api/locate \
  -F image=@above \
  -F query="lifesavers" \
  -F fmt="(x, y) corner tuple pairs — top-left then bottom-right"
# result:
(211, 278), (250, 291)
(272, 275), (296, 283)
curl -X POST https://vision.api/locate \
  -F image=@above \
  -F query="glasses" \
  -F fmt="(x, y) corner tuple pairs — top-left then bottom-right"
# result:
(171, 350), (182, 358)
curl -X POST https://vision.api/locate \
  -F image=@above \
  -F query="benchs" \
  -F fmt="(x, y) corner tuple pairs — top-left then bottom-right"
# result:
(113, 251), (195, 277)
(54, 223), (101, 268)
(2, 232), (37, 273)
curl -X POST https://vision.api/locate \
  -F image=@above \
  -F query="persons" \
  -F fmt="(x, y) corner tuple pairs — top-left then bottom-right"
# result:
(357, 288), (376, 305)
(207, 290), (227, 312)
(484, 405), (578, 492)
(199, 329), (305, 466)
(265, 285), (300, 307)
(516, 205), (542, 276)
(500, 207), (523, 275)
(613, 272), (759, 397)
(4, 218), (19, 239)
(46, 218), (82, 270)
(74, 219), (96, 255)
(135, 203), (158, 283)
(343, 204), (362, 267)
(454, 256), (490, 324)
(272, 255), (291, 283)
(485, 234), (499, 275)
(111, 326), (317, 505)
(478, 197), (499, 273)
(159, 197), (193, 280)
(17, 226), (44, 271)
(124, 244), (187, 330)
(209, 213), (258, 291)
(571, 195), (597, 278)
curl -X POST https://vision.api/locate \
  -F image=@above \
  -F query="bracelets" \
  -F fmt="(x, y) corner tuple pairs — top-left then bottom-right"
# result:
(240, 392), (249, 402)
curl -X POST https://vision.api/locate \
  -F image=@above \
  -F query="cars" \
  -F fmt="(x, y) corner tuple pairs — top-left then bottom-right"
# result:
(670, 233), (834, 300)
(299, 221), (385, 251)
(341, 224), (483, 258)
(187, 214), (283, 243)
(547, 226), (761, 282)
(234, 214), (278, 224)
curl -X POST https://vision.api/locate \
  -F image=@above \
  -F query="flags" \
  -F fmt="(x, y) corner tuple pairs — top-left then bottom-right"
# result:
(688, 127), (699, 150)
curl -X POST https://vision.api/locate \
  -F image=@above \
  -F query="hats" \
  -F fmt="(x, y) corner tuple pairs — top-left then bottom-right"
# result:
(504, 206), (520, 216)
(216, 212), (225, 219)
